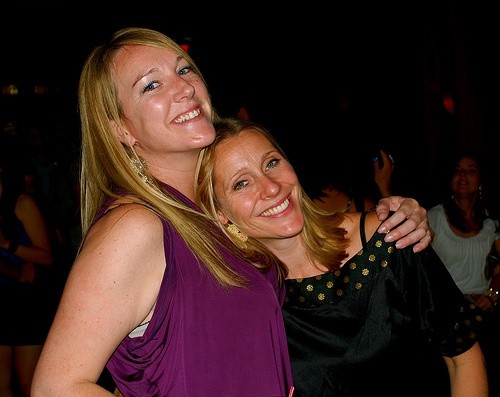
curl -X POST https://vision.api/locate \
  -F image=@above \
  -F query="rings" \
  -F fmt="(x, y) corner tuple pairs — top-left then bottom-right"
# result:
(392, 160), (394, 163)
(389, 154), (393, 160)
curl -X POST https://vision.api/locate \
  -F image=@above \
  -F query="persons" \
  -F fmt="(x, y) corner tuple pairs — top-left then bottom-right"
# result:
(0, 163), (51, 397)
(301, 149), (396, 215)
(197, 119), (490, 397)
(425, 155), (498, 314)
(30, 29), (433, 395)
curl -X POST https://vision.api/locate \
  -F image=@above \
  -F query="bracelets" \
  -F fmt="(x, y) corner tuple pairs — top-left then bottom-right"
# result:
(6, 240), (19, 253)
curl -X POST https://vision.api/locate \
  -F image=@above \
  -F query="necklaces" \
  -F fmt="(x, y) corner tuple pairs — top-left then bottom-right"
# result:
(345, 198), (352, 213)
